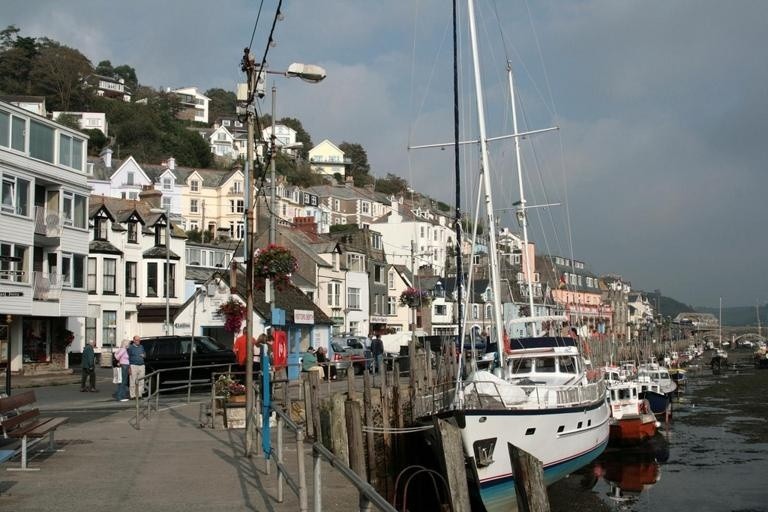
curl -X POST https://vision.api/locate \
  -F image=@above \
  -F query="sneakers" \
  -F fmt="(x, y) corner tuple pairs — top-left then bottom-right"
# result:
(79, 386), (99, 392)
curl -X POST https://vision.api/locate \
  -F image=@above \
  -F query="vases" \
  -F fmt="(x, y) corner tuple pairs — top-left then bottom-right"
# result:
(229, 395), (247, 404)
(408, 297), (420, 307)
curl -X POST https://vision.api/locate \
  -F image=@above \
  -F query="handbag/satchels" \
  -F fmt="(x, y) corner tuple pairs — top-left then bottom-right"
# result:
(112, 366), (123, 384)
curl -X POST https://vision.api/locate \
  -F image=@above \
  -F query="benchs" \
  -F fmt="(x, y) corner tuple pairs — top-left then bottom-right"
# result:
(0, 390), (71, 472)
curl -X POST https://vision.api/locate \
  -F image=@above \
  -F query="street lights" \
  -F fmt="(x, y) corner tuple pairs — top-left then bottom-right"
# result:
(151, 208), (169, 334)
(238, 63), (326, 456)
(409, 240), (432, 337)
(269, 84), (304, 335)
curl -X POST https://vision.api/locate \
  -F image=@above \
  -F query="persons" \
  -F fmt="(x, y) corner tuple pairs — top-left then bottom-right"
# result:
(365, 332), (384, 374)
(127, 336), (147, 400)
(568, 321), (599, 356)
(79, 340), (100, 391)
(113, 339), (129, 401)
(233, 326), (286, 400)
(302, 346), (337, 381)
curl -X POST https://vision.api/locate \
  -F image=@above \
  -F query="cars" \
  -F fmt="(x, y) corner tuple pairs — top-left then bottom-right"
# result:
(327, 335), (491, 376)
(127, 335), (260, 396)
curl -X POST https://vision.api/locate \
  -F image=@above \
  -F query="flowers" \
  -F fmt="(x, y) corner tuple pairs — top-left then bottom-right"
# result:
(217, 295), (247, 334)
(215, 375), (250, 396)
(62, 328), (75, 345)
(254, 241), (300, 291)
(399, 287), (433, 309)
